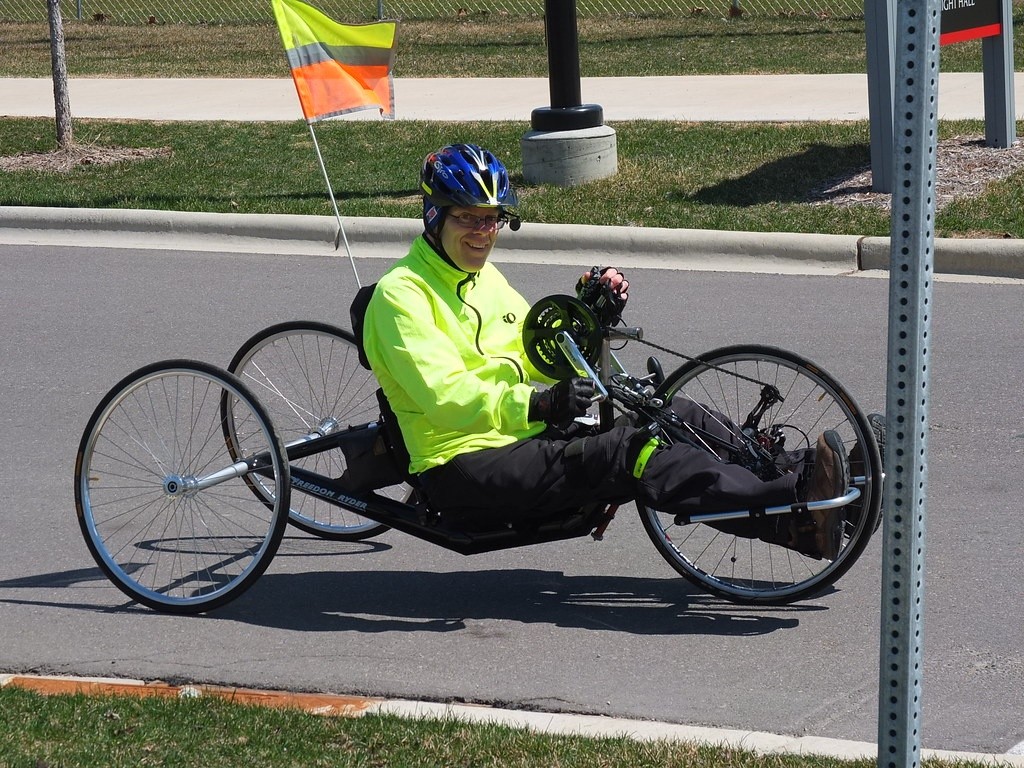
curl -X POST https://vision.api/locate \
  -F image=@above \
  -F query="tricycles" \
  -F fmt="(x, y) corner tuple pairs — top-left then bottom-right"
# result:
(73, 262), (884, 621)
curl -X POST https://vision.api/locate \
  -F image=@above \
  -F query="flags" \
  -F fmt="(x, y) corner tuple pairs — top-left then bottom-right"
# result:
(272, 0), (400, 127)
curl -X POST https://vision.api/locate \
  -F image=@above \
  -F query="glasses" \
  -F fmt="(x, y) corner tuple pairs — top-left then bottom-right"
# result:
(446, 212), (508, 230)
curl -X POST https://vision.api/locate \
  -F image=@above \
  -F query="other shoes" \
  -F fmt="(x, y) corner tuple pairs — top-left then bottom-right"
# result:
(804, 430), (850, 562)
(843, 414), (886, 539)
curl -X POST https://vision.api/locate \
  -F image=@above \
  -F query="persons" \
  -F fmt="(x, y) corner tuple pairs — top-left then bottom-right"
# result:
(362, 139), (892, 563)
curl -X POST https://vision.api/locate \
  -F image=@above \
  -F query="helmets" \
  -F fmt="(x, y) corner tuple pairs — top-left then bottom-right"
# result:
(422, 143), (517, 232)
